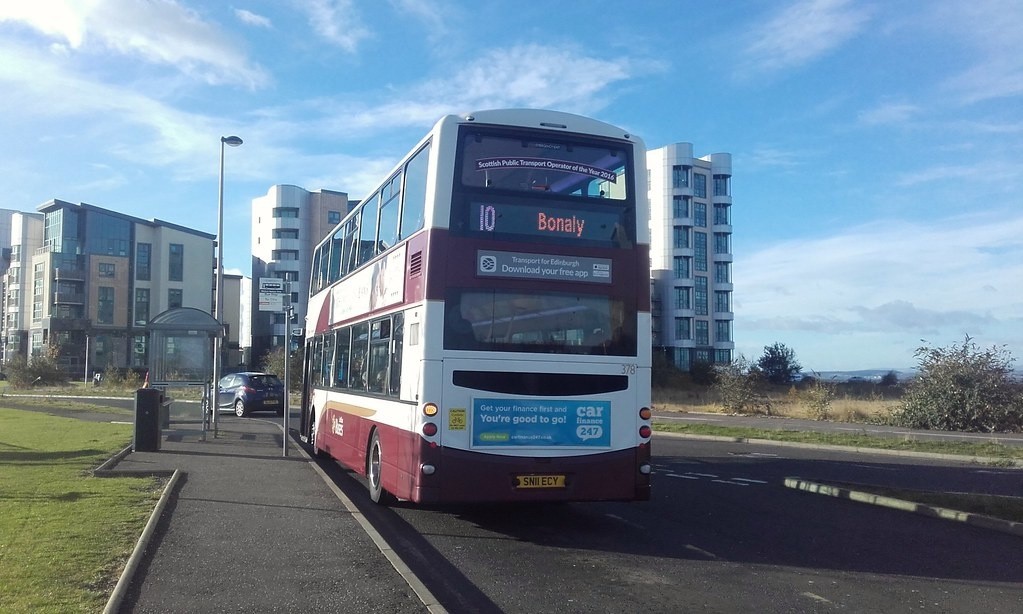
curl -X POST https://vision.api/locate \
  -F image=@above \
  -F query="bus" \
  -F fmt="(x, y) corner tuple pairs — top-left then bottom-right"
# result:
(301, 107), (652, 511)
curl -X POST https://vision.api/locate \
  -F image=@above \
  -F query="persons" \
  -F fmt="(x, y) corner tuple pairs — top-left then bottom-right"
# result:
(362, 370), (389, 393)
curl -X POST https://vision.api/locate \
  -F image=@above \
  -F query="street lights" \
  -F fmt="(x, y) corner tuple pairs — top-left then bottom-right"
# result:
(211, 136), (242, 438)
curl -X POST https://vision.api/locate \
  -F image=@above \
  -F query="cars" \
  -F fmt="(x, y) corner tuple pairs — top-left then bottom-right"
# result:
(202, 372), (286, 418)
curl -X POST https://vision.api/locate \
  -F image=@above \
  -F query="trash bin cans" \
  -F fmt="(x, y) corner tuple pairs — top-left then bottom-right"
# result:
(133, 388), (165, 451)
